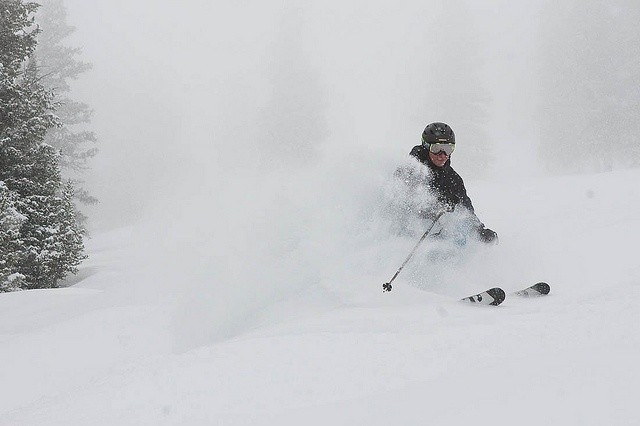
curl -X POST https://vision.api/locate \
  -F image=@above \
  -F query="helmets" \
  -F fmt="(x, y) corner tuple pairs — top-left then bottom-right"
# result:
(421, 123), (456, 148)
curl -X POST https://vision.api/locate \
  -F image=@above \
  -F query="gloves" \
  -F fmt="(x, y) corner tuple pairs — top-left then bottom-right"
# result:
(438, 193), (460, 212)
(481, 229), (497, 244)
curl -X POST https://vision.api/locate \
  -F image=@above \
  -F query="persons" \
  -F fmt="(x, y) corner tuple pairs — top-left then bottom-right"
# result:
(383, 122), (498, 245)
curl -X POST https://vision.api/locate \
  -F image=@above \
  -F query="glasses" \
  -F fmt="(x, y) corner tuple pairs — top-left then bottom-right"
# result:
(422, 138), (455, 156)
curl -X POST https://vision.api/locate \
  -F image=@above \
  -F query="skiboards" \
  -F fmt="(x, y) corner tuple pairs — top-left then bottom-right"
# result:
(462, 282), (550, 306)
